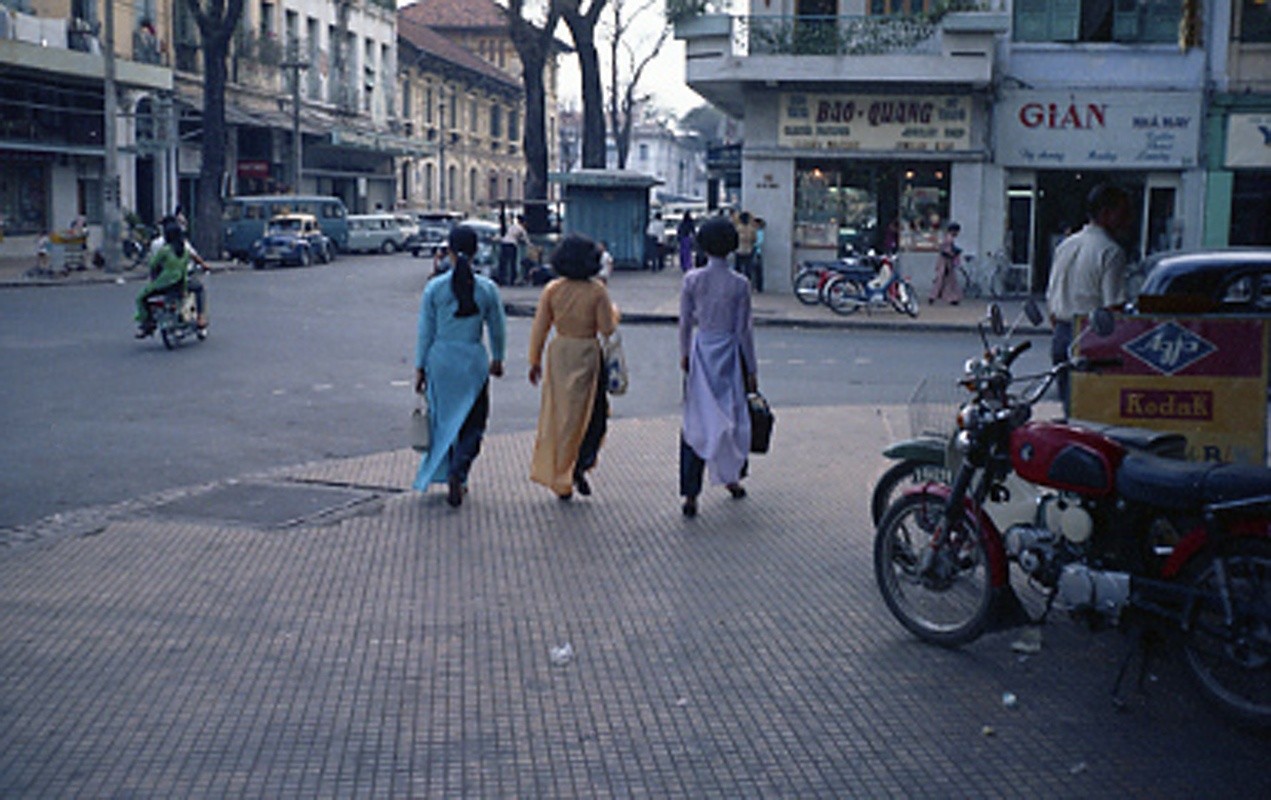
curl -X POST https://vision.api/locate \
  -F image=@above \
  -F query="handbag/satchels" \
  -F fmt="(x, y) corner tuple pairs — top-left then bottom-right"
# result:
(746, 391), (773, 453)
(409, 391), (432, 453)
(602, 328), (629, 396)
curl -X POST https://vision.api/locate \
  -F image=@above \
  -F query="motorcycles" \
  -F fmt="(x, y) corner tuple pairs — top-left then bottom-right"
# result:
(790, 217), (920, 319)
(874, 300), (1271, 720)
(870, 300), (1198, 574)
(148, 260), (214, 352)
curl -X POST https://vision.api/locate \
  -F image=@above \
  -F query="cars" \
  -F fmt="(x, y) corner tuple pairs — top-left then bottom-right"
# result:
(1066, 249), (1271, 466)
(250, 212), (335, 269)
(343, 212), (404, 255)
(406, 195), (750, 258)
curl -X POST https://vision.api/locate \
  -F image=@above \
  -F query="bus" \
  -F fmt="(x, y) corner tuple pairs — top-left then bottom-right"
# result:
(219, 193), (350, 264)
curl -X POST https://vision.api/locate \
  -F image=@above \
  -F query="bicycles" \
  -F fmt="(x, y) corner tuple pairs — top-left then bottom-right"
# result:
(115, 215), (161, 270)
(933, 248), (1020, 301)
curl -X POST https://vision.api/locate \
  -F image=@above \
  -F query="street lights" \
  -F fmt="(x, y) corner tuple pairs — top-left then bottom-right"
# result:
(65, 1), (120, 255)
(279, 58), (311, 195)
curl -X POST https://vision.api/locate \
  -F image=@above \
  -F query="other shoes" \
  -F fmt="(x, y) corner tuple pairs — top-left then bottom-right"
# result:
(446, 468), (744, 519)
(134, 312), (209, 340)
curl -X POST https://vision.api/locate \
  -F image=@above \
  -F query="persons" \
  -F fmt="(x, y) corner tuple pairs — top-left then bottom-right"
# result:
(927, 221), (963, 307)
(410, 223), (509, 506)
(526, 229), (622, 501)
(674, 212), (759, 518)
(133, 219), (191, 339)
(1044, 180), (1132, 419)
(150, 214), (213, 329)
(424, 202), (769, 297)
(174, 202), (190, 234)
